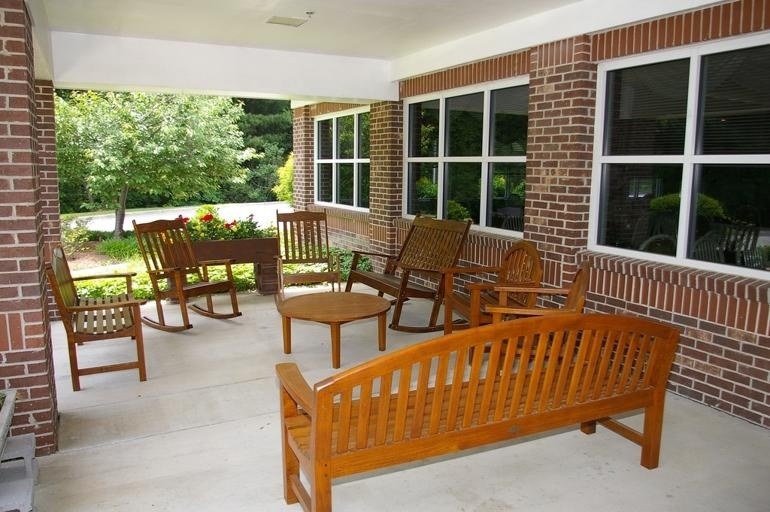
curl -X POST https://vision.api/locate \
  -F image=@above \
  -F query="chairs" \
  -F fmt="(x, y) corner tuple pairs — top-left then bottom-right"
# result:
(485, 260), (590, 325)
(346, 214), (477, 330)
(44, 214), (243, 391)
(274, 208), (342, 298)
(438, 241), (544, 338)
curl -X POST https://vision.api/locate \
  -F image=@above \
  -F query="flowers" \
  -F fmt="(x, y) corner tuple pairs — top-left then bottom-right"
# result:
(170, 204), (266, 241)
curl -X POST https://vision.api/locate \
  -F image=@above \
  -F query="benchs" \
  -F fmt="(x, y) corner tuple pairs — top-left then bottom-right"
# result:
(274, 312), (681, 512)
